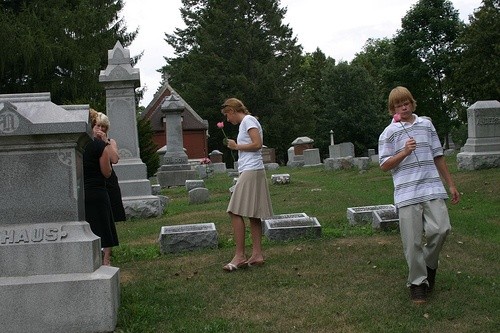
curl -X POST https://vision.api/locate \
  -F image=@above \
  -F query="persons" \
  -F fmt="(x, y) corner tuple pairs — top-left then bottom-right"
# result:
(82, 107), (128, 268)
(222, 98), (275, 272)
(378, 86), (461, 305)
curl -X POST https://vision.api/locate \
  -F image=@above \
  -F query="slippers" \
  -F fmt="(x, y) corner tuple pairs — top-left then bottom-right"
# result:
(222, 260), (264, 272)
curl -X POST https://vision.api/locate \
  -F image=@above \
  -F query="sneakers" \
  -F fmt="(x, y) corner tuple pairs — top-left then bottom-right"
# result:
(411, 267), (437, 304)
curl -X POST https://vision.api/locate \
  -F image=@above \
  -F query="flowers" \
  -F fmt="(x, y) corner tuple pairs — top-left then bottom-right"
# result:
(393, 113), (422, 169)
(217, 122), (236, 162)
